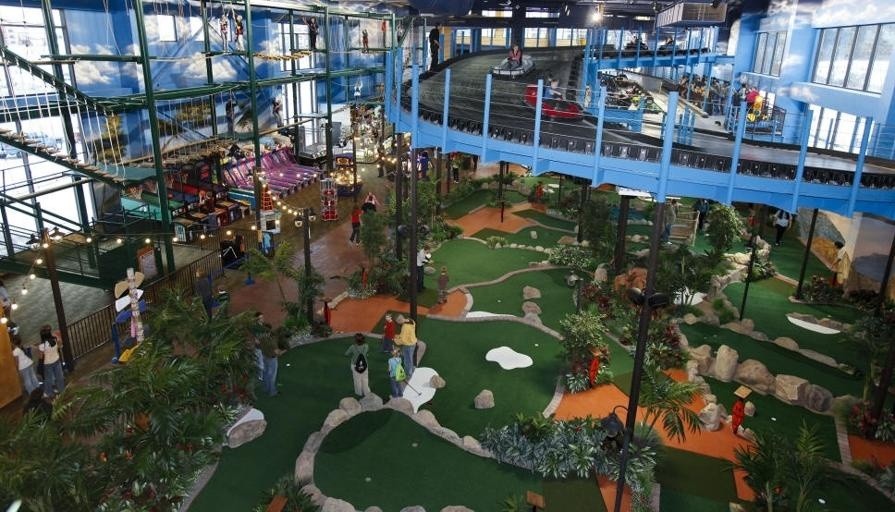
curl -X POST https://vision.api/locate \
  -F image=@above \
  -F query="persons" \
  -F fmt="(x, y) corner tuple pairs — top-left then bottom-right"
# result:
(347, 204), (361, 248)
(380, 312), (394, 352)
(418, 151), (471, 185)
(257, 322), (288, 397)
(192, 267), (214, 322)
(731, 398), (745, 433)
(664, 199), (680, 246)
(504, 45), (521, 70)
(361, 28), (369, 49)
(830, 240), (851, 288)
(391, 314), (415, 377)
(691, 198), (710, 235)
(679, 74), (765, 121)
(428, 22), (443, 74)
(437, 266), (450, 303)
(219, 15), (229, 51)
(528, 181), (544, 209)
(386, 348), (404, 399)
(544, 79), (563, 110)
(0, 279), (66, 402)
(416, 245), (431, 293)
(771, 208), (793, 248)
(360, 196), (377, 214)
(306, 17), (317, 51)
(251, 311), (269, 381)
(231, 15), (244, 51)
(343, 332), (370, 396)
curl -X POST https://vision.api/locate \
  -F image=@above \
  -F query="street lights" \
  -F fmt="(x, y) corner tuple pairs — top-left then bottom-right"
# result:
(28, 227), (81, 377)
(613, 285), (673, 510)
(293, 206), (322, 326)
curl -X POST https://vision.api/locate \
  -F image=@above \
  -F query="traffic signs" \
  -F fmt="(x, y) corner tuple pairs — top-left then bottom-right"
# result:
(114, 272), (145, 296)
(112, 289), (144, 312)
(115, 299), (147, 327)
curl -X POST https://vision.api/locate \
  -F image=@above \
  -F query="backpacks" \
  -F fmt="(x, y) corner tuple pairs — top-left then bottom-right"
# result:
(392, 358), (406, 383)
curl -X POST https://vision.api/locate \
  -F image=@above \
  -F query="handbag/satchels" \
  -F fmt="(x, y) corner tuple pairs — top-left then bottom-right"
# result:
(353, 343), (367, 374)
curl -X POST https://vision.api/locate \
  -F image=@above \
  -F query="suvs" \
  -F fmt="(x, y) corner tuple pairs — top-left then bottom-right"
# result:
(0, 131), (62, 160)
